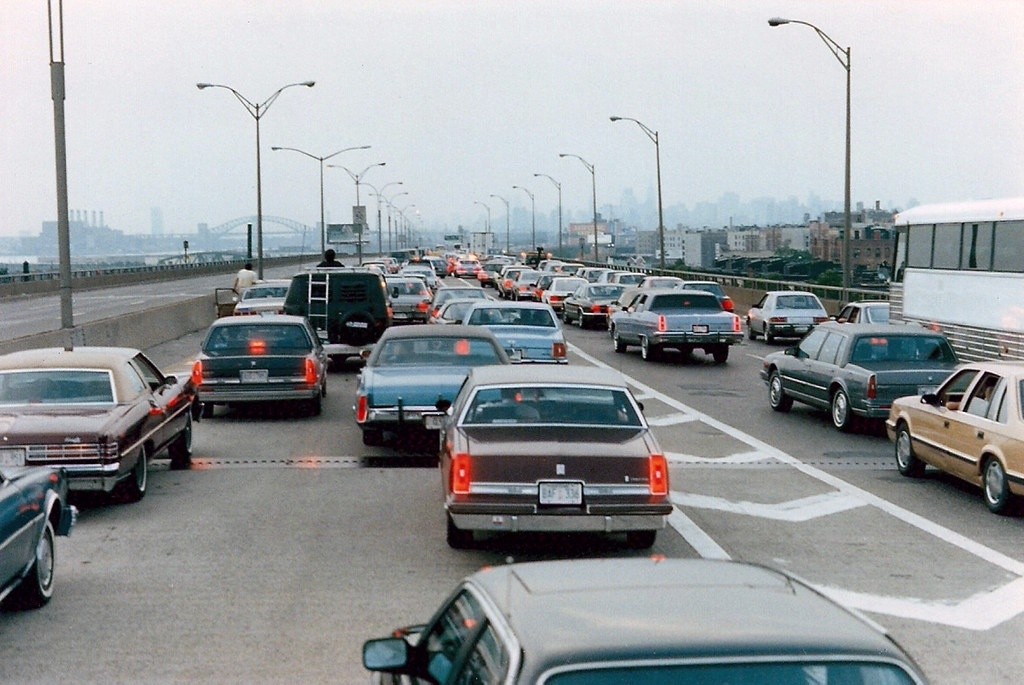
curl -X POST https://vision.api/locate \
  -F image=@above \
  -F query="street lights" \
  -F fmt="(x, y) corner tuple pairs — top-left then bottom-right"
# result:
(326, 161), (386, 267)
(560, 152), (599, 263)
(475, 201), (491, 250)
(357, 180), (403, 261)
(268, 145), (376, 270)
(368, 190), (409, 251)
(766, 15), (853, 313)
(194, 79), (313, 284)
(609, 115), (669, 273)
(535, 173), (563, 256)
(490, 194), (509, 253)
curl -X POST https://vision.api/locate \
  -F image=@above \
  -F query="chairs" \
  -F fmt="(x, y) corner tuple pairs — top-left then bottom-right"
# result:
(887, 338), (908, 359)
(857, 343), (873, 360)
(479, 314), (490, 322)
(919, 343), (942, 361)
(224, 326), (246, 347)
(483, 407), (515, 420)
(851, 312), (858, 322)
(795, 297), (808, 309)
(443, 293), (453, 303)
(610, 290), (618, 296)
(395, 340), (417, 363)
(272, 326), (300, 347)
(513, 310), (532, 325)
(571, 403), (617, 423)
(410, 287), (421, 295)
(527, 311), (548, 326)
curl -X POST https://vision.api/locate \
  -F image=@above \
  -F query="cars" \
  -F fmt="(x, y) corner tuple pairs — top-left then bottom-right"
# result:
(416, 362), (682, 545)
(192, 313), (344, 417)
(680, 281), (737, 313)
(744, 291), (832, 346)
(828, 300), (889, 326)
(884, 361), (1024, 513)
(0, 344), (205, 505)
(757, 321), (963, 434)
(210, 282), (294, 319)
(608, 288), (747, 363)
(0, 462), (78, 620)
(361, 242), (683, 339)
(358, 558), (926, 685)
(354, 326), (528, 448)
(459, 300), (582, 368)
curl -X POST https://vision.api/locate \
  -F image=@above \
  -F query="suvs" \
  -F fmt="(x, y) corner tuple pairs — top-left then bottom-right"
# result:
(282, 265), (401, 366)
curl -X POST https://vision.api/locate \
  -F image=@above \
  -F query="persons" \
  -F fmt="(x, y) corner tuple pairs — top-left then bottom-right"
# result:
(946, 377), (998, 410)
(468, 389), (540, 420)
(317, 249), (345, 267)
(447, 257), (454, 276)
(234, 263), (259, 289)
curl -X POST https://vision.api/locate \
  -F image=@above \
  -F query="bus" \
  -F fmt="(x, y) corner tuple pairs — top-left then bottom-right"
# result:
(867, 200), (1024, 362)
